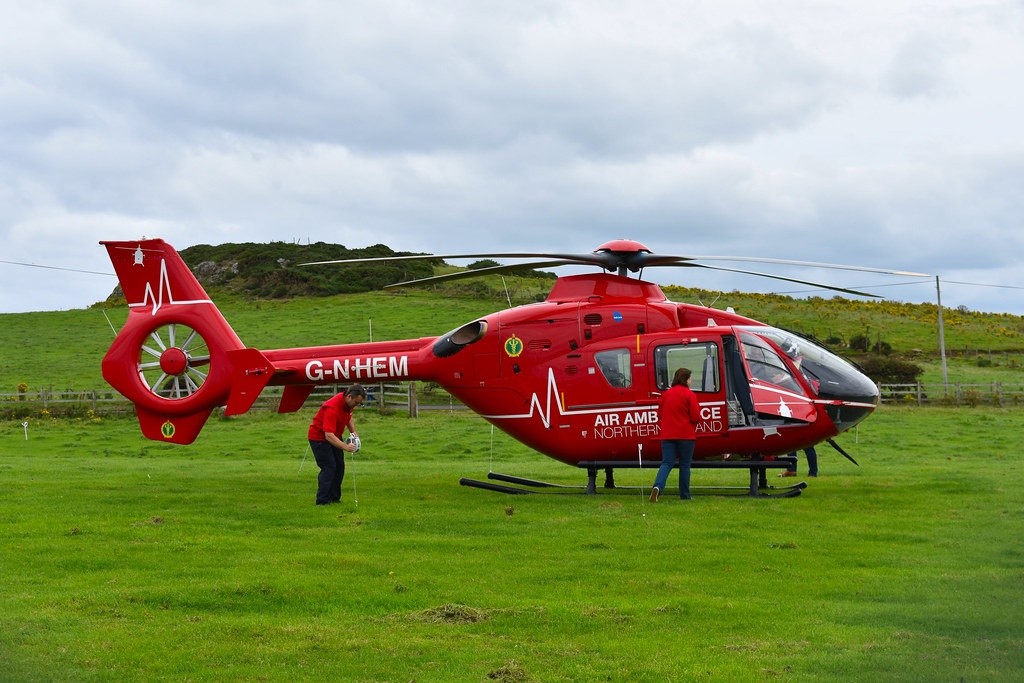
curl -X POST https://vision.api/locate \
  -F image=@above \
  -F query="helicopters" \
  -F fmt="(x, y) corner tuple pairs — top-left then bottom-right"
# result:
(95, 239), (937, 513)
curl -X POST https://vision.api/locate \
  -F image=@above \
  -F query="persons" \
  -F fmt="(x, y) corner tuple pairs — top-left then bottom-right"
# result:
(308, 385), (366, 505)
(777, 446), (818, 477)
(361, 387), (380, 407)
(649, 368), (701, 502)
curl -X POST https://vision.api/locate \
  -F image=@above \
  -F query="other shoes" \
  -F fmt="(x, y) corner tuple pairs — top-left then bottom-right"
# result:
(649, 487), (659, 502)
(777, 471), (797, 477)
